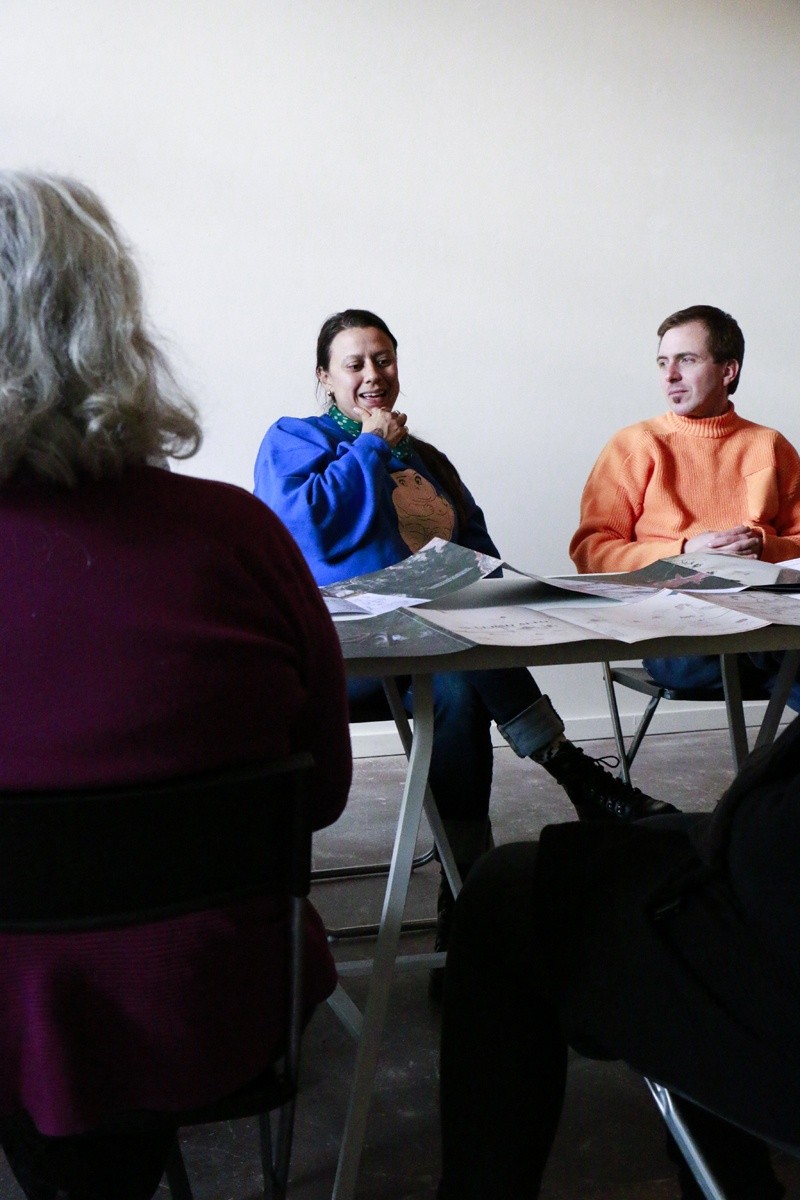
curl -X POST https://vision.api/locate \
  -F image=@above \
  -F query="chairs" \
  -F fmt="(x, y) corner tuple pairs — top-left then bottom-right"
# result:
(603, 658), (771, 786)
(1, 753), (350, 1200)
(310, 677), (440, 938)
(639, 1070), (799, 1199)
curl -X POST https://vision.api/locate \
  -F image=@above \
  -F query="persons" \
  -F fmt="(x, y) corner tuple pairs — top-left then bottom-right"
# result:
(0, 172), (347, 1200)
(432, 718), (800, 1199)
(256, 309), (682, 913)
(569, 303), (798, 713)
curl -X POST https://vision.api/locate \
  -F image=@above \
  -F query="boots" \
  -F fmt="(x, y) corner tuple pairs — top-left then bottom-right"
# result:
(545, 739), (682, 822)
(436, 814), (494, 939)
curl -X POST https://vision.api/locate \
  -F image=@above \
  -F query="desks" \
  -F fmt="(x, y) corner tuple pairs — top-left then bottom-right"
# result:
(326, 624), (800, 1200)
